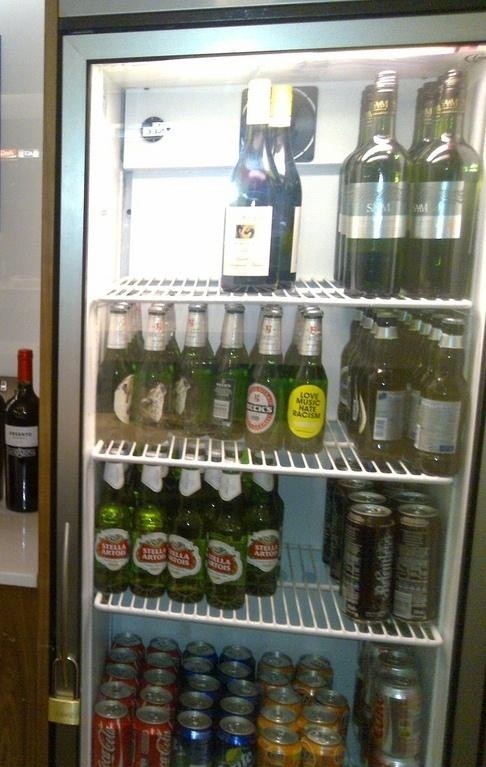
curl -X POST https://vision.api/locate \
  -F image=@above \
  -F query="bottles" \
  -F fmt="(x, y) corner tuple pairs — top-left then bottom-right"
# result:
(4, 349), (39, 513)
(220, 79), (280, 295)
(401, 67), (482, 298)
(268, 85), (302, 291)
(94, 447), (285, 610)
(96, 301), (471, 477)
(335, 69), (414, 295)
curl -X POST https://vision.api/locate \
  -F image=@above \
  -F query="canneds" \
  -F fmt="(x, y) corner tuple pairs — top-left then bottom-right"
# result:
(91, 631), (177, 767)
(324, 477), (439, 623)
(352, 642), (428, 766)
(173, 642), (259, 767)
(256, 654), (347, 766)
